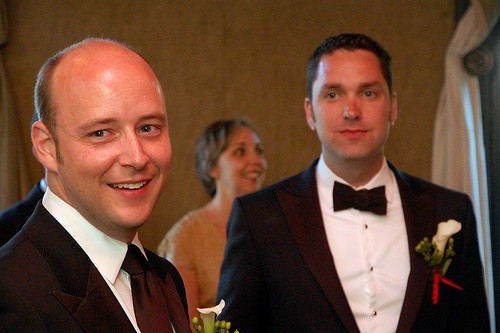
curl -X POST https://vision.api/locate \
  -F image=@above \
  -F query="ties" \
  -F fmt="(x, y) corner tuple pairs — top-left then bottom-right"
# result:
(120, 243), (173, 333)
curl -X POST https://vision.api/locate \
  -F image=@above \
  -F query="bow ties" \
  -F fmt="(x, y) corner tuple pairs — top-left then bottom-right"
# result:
(333, 181), (387, 215)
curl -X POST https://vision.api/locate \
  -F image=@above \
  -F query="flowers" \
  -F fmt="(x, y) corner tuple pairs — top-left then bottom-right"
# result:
(415, 219), (462, 303)
(191, 299), (239, 333)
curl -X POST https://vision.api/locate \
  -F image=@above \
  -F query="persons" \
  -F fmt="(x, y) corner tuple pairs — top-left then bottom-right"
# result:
(215, 33), (492, 333)
(157, 118), (268, 333)
(0, 37), (190, 333)
